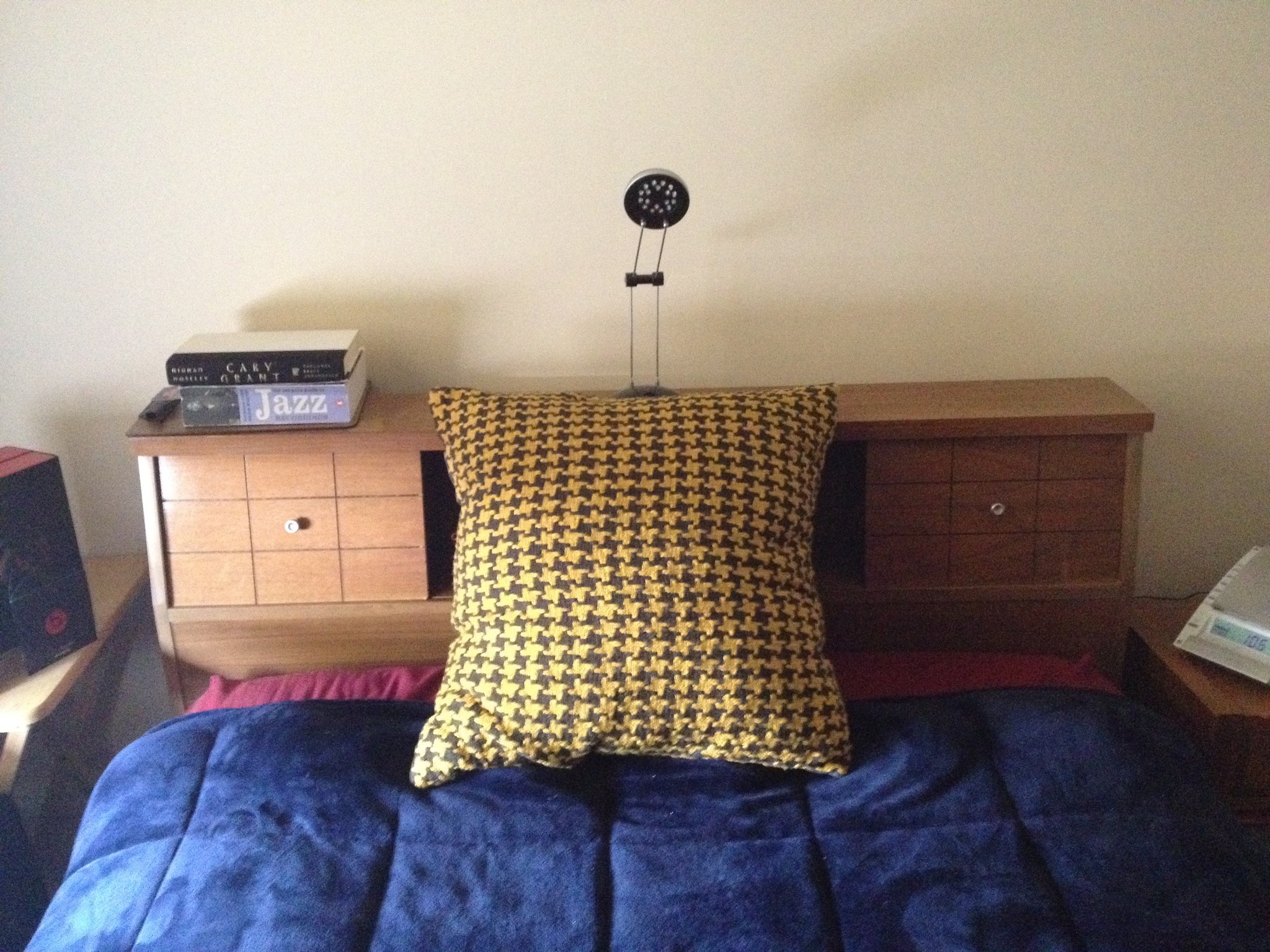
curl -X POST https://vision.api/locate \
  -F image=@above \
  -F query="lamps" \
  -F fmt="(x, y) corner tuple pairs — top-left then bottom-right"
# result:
(616, 166), (691, 400)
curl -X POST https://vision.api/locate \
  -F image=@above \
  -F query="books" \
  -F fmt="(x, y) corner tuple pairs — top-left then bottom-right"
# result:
(179, 354), (367, 426)
(166, 329), (362, 386)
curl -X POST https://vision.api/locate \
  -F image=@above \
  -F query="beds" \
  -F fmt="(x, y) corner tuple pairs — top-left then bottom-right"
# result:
(22, 370), (1270, 952)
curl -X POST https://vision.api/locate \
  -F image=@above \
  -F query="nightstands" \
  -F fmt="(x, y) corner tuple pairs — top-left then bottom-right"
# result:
(0, 550), (156, 916)
(1122, 599), (1270, 847)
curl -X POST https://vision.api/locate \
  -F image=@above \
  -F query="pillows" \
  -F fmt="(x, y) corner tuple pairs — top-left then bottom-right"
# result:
(411, 381), (855, 790)
